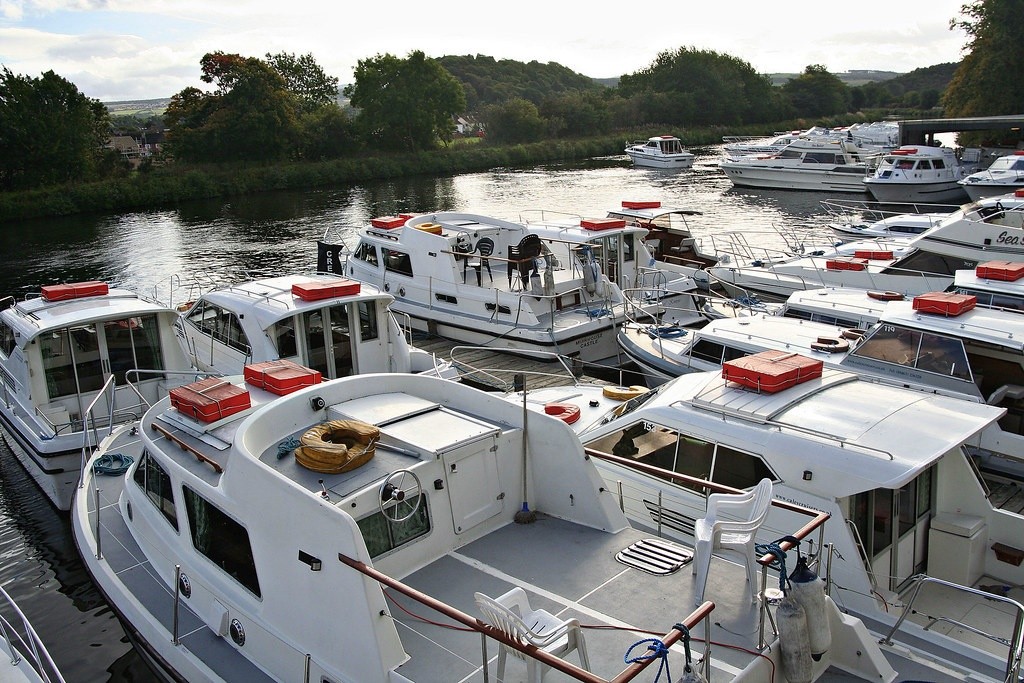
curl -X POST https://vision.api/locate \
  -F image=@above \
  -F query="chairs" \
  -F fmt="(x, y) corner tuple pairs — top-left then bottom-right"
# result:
(464, 237), (495, 287)
(508, 234), (542, 291)
(474, 587), (591, 683)
(693, 478), (773, 607)
(671, 238), (695, 252)
(645, 238), (660, 258)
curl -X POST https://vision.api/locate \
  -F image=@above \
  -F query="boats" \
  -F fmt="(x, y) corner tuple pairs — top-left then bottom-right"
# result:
(519, 209), (707, 327)
(70, 359), (1024, 683)
(700, 265), (1024, 330)
(803, 121), (899, 152)
(709, 189), (1024, 299)
(718, 139), (879, 192)
(151, 271), (462, 382)
(862, 145), (978, 205)
(624, 136), (694, 169)
(957, 151), (1024, 201)
(819, 198), (952, 243)
(606, 200), (721, 282)
(346, 212), (666, 362)
(616, 288), (1024, 479)
(722, 131), (801, 156)
(0, 280), (210, 510)
(449, 345), (1024, 683)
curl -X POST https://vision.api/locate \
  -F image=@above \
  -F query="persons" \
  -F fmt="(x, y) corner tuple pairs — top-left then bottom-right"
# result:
(845, 130), (853, 142)
